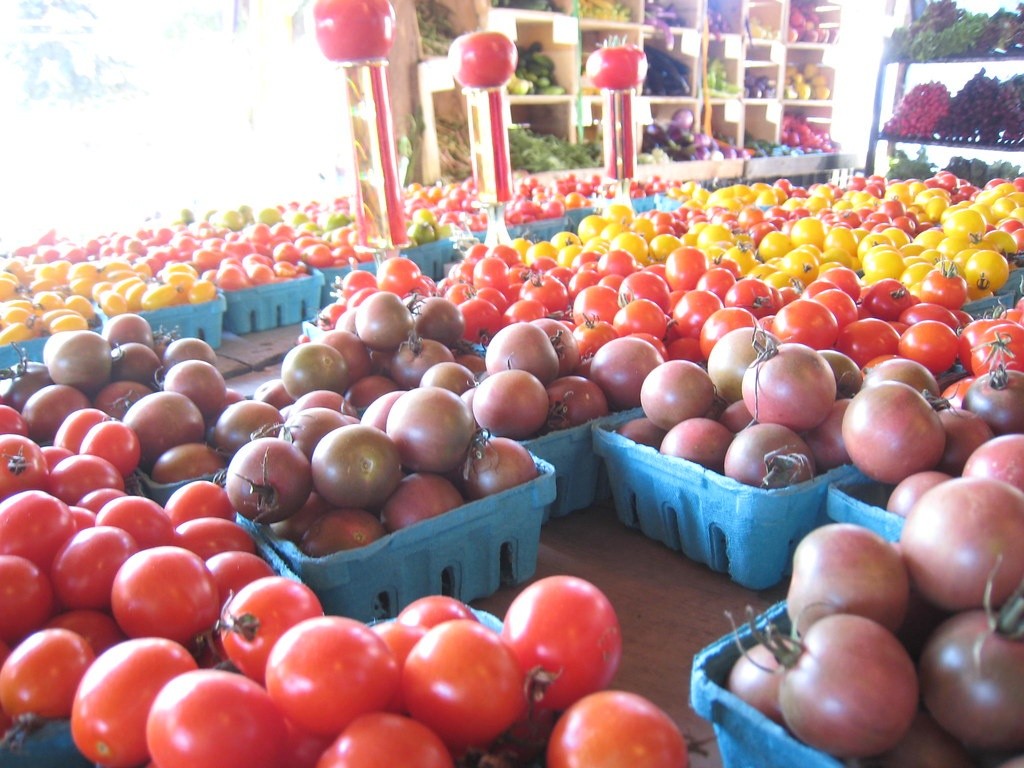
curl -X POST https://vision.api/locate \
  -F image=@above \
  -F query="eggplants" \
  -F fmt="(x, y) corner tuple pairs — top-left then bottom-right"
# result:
(642, 0), (753, 160)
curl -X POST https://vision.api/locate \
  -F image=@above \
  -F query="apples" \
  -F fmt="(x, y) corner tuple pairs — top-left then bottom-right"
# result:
(778, 0), (838, 153)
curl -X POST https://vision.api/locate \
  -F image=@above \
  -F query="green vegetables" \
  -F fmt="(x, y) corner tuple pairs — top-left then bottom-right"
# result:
(889, 0), (1024, 60)
(506, 123), (602, 170)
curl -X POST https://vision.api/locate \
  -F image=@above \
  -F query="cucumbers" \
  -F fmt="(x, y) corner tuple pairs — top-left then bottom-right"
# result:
(504, 42), (566, 95)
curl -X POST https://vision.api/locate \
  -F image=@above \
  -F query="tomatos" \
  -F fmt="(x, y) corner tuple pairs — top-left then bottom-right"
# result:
(585, 34), (648, 90)
(313, 0), (397, 62)
(447, 27), (518, 90)
(0, 171), (1024, 768)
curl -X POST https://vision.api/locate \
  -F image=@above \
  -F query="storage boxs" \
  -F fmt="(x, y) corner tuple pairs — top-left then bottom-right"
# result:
(631, 197), (655, 213)
(653, 193), (681, 211)
(0, 308), (104, 369)
(472, 226), (519, 243)
(0, 521), (302, 768)
(591, 408), (858, 590)
(401, 237), (465, 282)
(566, 207), (593, 234)
(522, 216), (571, 243)
(218, 265), (325, 335)
(318, 253), (408, 310)
(689, 599), (845, 768)
(961, 288), (1016, 319)
(98, 293), (228, 350)
(823, 473), (907, 542)
(253, 451), (558, 622)
(485, 424), (612, 526)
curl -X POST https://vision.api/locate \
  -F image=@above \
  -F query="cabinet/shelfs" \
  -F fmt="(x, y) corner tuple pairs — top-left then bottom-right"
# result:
(864, 35), (1024, 176)
(342, 0), (859, 186)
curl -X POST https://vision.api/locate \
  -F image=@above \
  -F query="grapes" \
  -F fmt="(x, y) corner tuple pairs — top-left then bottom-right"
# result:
(881, 73), (1024, 142)
